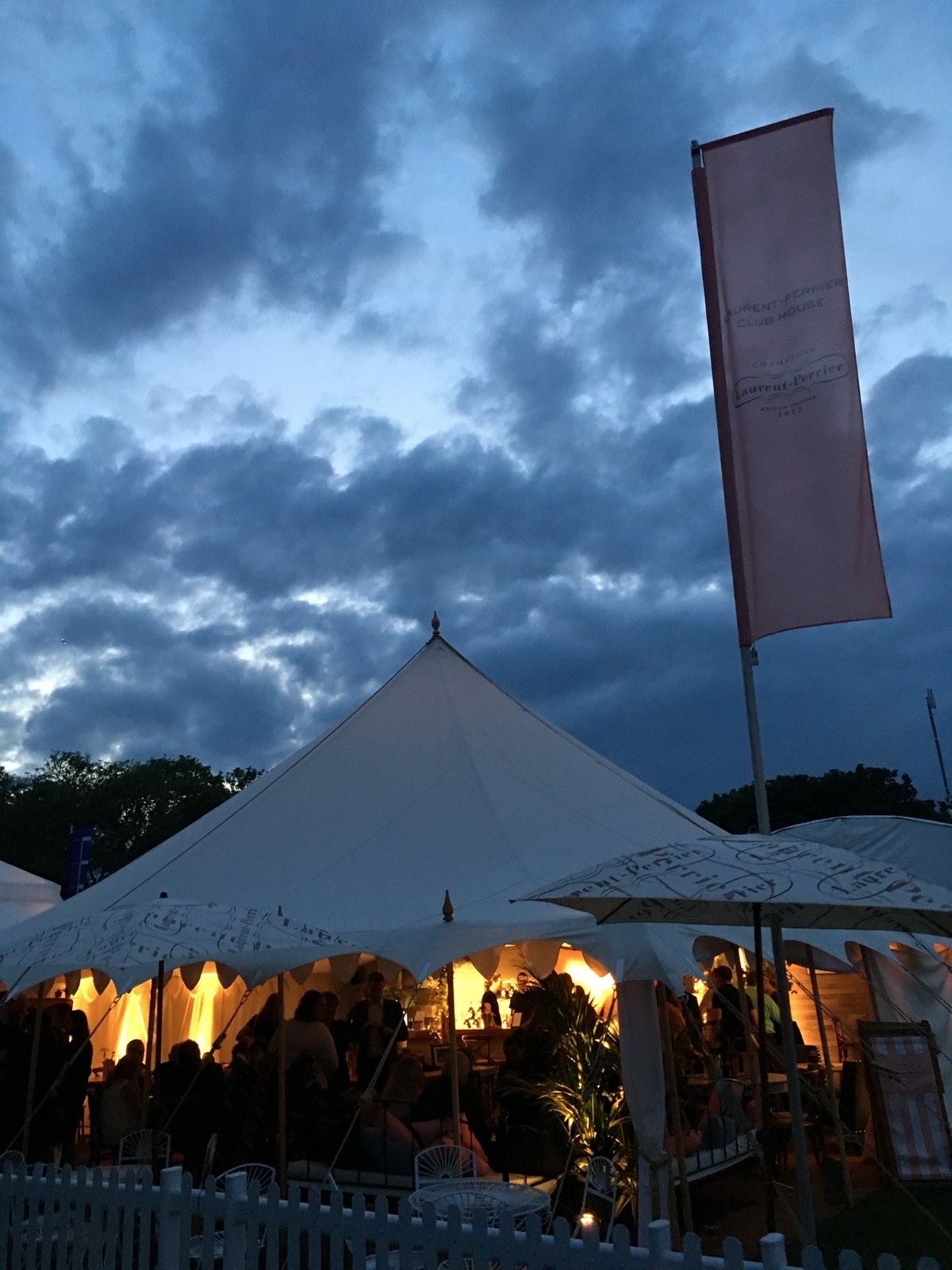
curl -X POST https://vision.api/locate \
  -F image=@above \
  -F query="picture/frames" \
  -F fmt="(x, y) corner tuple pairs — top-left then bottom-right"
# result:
(432, 1045), (450, 1068)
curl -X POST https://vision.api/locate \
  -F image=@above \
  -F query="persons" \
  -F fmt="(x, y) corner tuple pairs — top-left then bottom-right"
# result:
(106, 1040), (152, 1120)
(100, 1060), (143, 1164)
(144, 966), (805, 1239)
(0, 990), (93, 1176)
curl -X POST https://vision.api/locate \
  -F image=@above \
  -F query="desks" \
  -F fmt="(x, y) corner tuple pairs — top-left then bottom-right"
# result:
(408, 1180), (551, 1232)
(57, 1164), (149, 1183)
(423, 1066), (497, 1113)
(687, 1062), (823, 1096)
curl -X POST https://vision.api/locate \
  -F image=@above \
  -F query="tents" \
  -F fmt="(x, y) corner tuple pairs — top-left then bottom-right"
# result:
(0, 607), (879, 1229)
(766, 815), (952, 1122)
(0, 858), (65, 931)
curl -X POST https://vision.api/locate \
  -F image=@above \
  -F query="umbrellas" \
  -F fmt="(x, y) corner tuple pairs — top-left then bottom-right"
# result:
(508, 824), (952, 1234)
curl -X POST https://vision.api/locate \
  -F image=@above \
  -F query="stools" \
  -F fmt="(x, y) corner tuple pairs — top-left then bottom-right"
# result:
(762, 1077), (837, 1218)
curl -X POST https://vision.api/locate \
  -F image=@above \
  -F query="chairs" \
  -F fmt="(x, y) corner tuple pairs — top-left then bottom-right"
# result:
(414, 1145), (477, 1219)
(189, 1163), (276, 1270)
(118, 1129), (170, 1167)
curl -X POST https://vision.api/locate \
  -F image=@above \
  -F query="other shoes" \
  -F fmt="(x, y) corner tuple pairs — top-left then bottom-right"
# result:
(749, 1167), (776, 1184)
